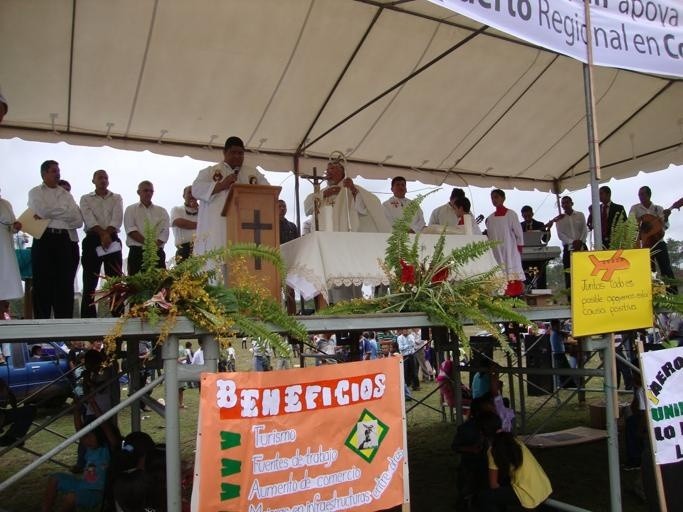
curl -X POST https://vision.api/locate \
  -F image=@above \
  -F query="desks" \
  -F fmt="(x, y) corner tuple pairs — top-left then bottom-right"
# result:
(279, 231), (487, 322)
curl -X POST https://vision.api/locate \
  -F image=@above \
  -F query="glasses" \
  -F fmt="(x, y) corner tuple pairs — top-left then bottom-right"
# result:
(185, 208), (198, 215)
(139, 189), (154, 192)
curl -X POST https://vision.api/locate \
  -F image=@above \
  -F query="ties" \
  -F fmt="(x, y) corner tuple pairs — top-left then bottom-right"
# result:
(601, 205), (607, 240)
(527, 224), (530, 230)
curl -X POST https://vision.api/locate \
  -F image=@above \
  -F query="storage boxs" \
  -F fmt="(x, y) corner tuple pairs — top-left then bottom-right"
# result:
(516, 425), (609, 492)
(589, 400), (632, 429)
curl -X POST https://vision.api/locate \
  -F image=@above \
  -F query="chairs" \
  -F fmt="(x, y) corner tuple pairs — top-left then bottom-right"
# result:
(434, 375), (471, 424)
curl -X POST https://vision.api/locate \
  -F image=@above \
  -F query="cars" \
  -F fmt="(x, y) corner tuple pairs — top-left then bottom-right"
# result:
(0, 340), (128, 413)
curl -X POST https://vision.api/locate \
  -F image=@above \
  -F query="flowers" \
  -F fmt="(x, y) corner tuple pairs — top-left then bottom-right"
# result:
(314, 188), (540, 358)
(91, 218), (311, 360)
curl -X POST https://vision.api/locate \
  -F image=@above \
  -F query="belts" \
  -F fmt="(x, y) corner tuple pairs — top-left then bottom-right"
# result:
(46, 228), (68, 234)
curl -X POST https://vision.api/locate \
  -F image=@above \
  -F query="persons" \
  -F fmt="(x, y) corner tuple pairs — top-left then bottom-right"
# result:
(0, 160), (170, 319)
(171, 137), (426, 305)
(429, 185), (679, 295)
(1, 313), (683, 512)
(1, 97), (9, 122)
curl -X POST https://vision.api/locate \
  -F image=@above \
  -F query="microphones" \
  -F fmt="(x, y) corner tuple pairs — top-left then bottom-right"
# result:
(234, 167), (240, 174)
